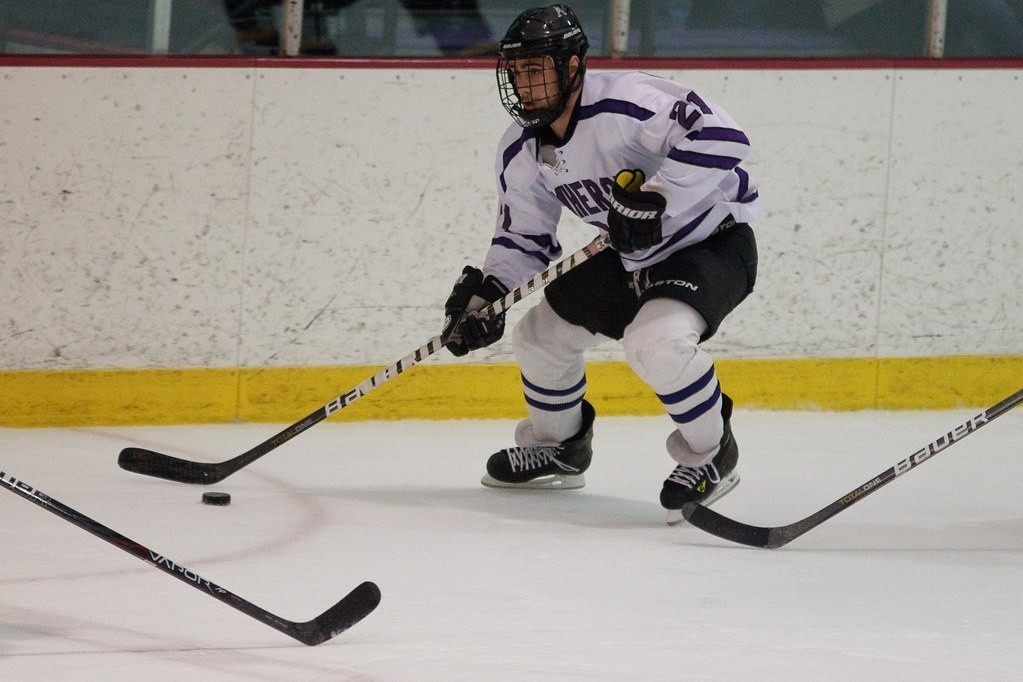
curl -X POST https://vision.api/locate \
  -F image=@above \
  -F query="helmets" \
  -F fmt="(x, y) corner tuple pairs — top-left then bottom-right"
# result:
(495, 3), (590, 131)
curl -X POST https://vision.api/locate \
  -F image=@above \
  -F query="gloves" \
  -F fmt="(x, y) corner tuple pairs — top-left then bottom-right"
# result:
(606, 169), (667, 254)
(443, 265), (507, 357)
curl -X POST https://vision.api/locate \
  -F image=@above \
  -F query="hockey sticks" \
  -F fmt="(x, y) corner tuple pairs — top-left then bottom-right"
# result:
(115, 232), (612, 487)
(0, 469), (384, 649)
(677, 388), (1022, 551)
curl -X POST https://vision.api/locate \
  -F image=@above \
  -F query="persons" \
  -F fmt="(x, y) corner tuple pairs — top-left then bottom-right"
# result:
(445, 2), (761, 510)
(220, 0), (500, 62)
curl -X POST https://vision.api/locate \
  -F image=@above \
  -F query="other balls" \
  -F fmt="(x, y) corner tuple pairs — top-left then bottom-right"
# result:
(201, 491), (232, 507)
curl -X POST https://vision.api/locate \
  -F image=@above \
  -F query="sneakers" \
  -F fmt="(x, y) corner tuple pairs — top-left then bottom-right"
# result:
(481, 399), (596, 489)
(658, 391), (741, 525)
